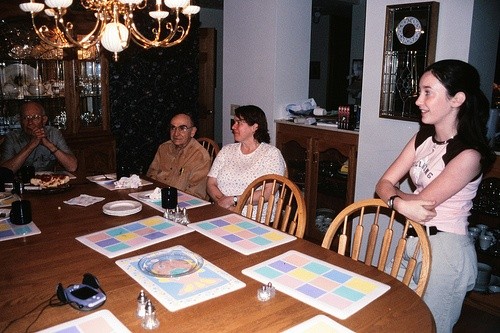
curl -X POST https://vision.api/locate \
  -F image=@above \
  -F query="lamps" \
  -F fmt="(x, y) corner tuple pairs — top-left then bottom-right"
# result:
(19, 0), (200, 61)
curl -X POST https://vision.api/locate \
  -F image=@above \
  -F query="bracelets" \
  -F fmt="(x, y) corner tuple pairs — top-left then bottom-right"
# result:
(50, 148), (59, 154)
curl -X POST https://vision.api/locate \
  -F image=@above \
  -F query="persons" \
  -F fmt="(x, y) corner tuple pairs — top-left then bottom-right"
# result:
(206, 105), (288, 227)
(376, 59), (496, 333)
(145, 114), (211, 201)
(0, 102), (78, 172)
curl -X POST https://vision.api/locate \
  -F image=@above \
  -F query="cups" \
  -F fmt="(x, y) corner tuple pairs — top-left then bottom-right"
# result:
(314, 215), (333, 233)
(162, 187), (177, 209)
(116, 165), (130, 181)
(11, 200), (32, 225)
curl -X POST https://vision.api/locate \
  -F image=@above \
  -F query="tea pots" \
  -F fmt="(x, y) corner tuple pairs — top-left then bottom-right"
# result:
(1, 80), (51, 98)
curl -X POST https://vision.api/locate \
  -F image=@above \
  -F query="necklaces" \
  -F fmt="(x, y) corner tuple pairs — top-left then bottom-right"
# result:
(433, 136), (454, 152)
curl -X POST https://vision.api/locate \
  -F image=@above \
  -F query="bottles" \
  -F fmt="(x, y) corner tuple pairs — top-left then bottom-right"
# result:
(137, 290), (160, 330)
(467, 224), (500, 294)
(163, 204), (190, 225)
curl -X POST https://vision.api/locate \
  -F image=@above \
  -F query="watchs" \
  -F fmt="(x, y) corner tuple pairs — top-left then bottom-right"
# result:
(387, 195), (403, 209)
(233, 196), (237, 207)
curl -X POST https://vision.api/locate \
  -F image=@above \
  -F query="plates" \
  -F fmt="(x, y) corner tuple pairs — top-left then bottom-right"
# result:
(138, 248), (204, 277)
(0, 191), (21, 207)
(102, 199), (142, 216)
(90, 175), (117, 184)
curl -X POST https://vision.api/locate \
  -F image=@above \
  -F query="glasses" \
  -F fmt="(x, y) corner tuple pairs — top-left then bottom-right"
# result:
(169, 124), (192, 131)
(22, 114), (45, 120)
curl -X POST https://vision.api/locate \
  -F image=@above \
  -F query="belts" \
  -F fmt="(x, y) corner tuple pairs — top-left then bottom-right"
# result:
(406, 226), (440, 238)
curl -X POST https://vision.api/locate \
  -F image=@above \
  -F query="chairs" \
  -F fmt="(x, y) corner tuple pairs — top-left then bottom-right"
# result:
(189, 138), (222, 206)
(322, 199), (432, 299)
(235, 174), (306, 241)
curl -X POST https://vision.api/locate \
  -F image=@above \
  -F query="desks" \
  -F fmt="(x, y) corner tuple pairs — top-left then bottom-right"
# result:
(0, 166), (438, 333)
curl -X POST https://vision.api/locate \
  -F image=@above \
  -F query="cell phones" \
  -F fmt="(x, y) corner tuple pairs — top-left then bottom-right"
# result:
(64, 283), (107, 312)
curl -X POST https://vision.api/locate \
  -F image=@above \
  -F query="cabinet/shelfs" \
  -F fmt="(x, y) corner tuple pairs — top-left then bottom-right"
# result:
(274, 119), (359, 258)
(0, 0), (118, 177)
(463, 156), (500, 316)
